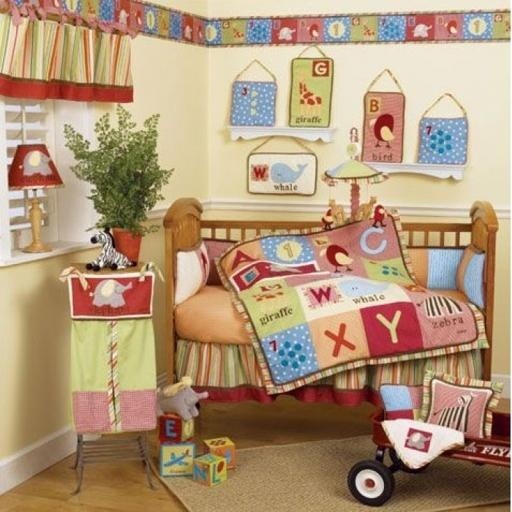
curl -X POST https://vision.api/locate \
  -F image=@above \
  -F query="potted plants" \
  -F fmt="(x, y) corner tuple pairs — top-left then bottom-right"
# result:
(64, 104), (176, 267)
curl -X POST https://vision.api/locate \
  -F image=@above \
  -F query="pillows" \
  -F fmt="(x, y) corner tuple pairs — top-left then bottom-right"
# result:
(173, 238), (209, 314)
(377, 369), (503, 443)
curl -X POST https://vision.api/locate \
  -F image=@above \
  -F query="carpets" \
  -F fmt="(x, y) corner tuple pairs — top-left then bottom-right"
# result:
(148, 434), (510, 512)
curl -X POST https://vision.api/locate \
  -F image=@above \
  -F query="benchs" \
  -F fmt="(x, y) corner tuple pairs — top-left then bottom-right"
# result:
(158, 196), (501, 388)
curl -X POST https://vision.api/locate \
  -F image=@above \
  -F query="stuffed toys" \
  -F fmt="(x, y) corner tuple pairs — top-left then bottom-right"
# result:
(86, 231), (137, 272)
(160, 376), (208, 420)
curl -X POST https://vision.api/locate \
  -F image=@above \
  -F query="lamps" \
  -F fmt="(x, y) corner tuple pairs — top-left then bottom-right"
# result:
(9, 144), (64, 254)
